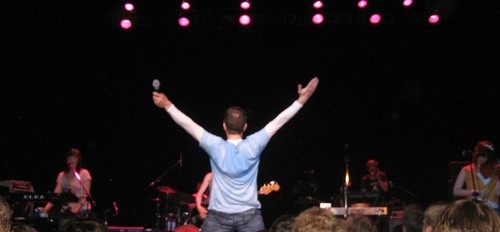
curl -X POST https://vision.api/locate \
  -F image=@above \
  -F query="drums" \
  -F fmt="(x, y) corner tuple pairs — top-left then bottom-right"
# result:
(162, 212), (178, 231)
(168, 203), (188, 225)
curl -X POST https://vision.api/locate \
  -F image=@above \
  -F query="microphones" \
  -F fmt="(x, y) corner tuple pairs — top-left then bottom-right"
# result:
(69, 161), (74, 167)
(180, 152), (183, 168)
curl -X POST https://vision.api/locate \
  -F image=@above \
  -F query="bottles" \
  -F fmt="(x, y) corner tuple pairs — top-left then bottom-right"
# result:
(166, 213), (176, 232)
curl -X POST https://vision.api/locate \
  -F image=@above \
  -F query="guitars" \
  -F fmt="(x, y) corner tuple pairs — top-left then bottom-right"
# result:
(192, 179), (281, 228)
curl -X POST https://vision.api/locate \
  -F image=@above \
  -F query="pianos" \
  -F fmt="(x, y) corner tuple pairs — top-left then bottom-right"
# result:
(3, 190), (108, 226)
(330, 189), (388, 229)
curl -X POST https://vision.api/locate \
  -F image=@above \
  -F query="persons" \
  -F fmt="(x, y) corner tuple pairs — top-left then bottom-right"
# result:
(0, 195), (43, 232)
(270, 207), (377, 232)
(402, 198), (500, 232)
(150, 158), (200, 215)
(52, 212), (107, 232)
(453, 141), (500, 215)
(153, 78), (319, 232)
(196, 172), (213, 218)
(37, 148), (92, 229)
(362, 160), (393, 207)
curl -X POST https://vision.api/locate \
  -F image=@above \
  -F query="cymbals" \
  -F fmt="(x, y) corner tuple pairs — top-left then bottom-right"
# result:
(157, 186), (176, 193)
